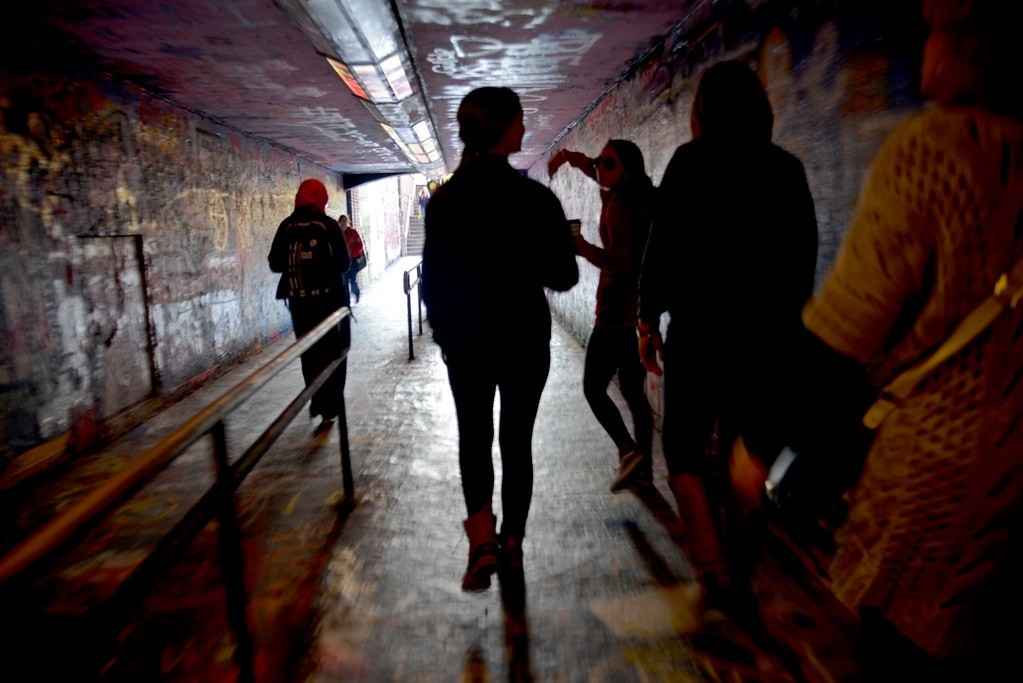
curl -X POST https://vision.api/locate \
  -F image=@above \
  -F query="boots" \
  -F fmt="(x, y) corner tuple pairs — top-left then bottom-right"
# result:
(462, 511), (525, 611)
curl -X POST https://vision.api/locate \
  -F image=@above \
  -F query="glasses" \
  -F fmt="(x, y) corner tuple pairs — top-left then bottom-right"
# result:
(592, 156), (623, 171)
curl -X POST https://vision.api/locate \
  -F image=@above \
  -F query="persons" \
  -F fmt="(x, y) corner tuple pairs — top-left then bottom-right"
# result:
(268, 0), (1023, 682)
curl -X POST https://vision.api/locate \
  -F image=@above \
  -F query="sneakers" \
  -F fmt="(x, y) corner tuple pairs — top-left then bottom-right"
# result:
(610, 443), (653, 494)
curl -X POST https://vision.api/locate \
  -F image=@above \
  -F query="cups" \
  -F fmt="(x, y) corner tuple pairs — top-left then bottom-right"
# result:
(569, 218), (581, 236)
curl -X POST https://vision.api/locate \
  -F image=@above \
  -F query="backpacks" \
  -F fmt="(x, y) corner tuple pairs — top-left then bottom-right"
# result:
(285, 215), (334, 297)
